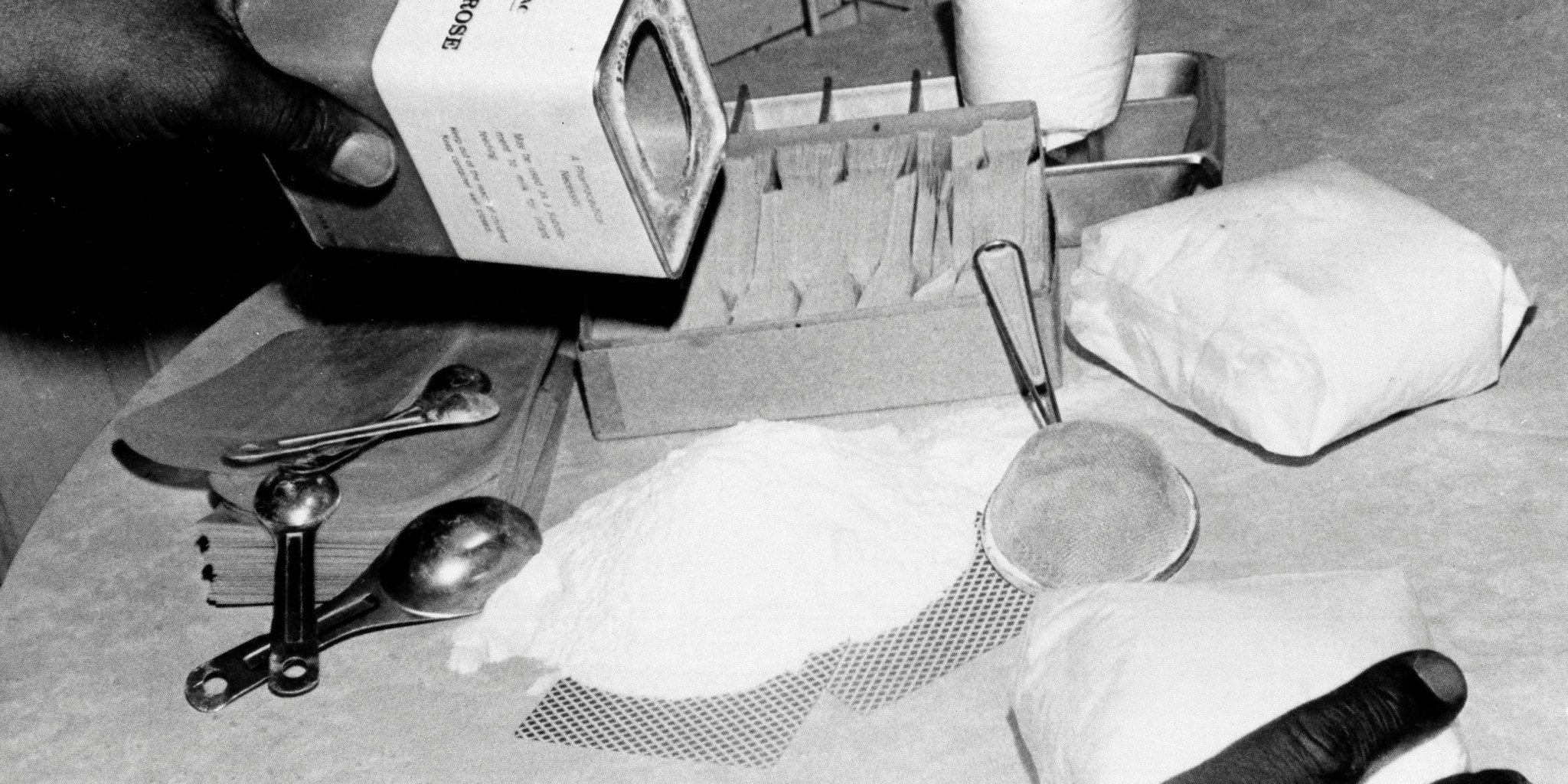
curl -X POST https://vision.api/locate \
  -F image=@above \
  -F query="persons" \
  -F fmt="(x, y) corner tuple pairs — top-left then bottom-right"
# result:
(1154, 647), (1538, 784)
(1, 0), (400, 191)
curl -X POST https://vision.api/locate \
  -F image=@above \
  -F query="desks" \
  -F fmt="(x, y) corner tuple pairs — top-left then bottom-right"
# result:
(2, 2), (1568, 784)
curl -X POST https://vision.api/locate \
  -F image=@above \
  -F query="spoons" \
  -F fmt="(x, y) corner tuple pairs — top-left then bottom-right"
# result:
(184, 365), (544, 715)
